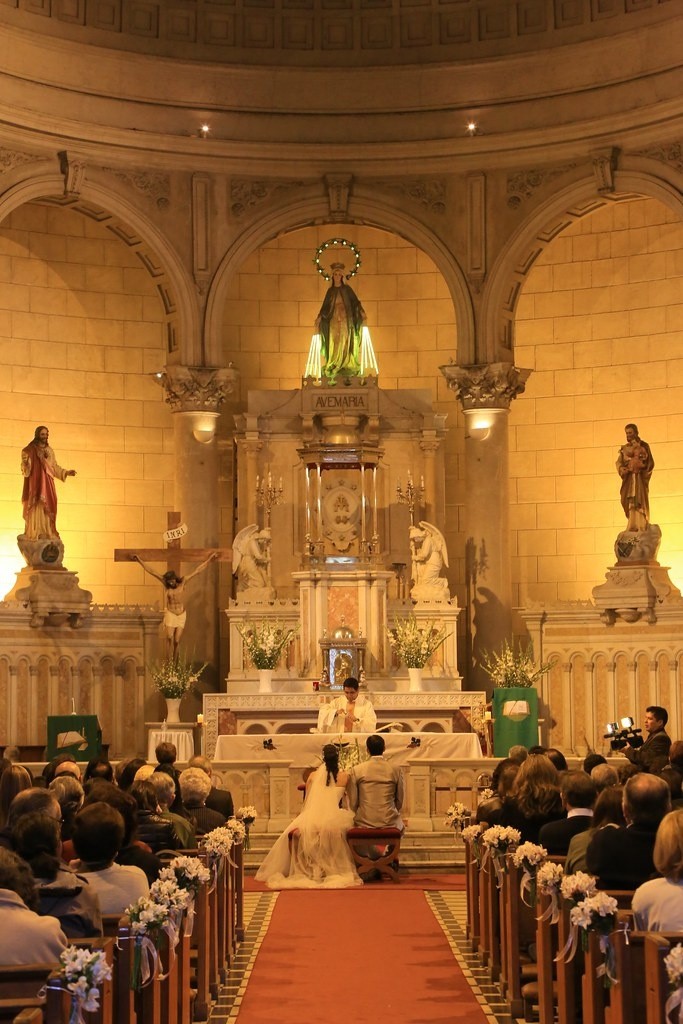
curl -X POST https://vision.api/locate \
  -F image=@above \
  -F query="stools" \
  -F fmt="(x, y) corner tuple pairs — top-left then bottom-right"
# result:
(101, 744), (109, 759)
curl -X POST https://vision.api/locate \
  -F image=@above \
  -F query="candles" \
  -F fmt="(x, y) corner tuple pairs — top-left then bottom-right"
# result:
(410, 475), (414, 488)
(279, 477), (283, 490)
(197, 713), (203, 723)
(421, 474), (424, 489)
(485, 710), (491, 720)
(256, 474), (259, 487)
(407, 470), (411, 482)
(268, 472), (271, 488)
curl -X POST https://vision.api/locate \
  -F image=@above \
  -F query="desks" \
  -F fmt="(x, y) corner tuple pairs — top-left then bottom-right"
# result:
(212, 732), (483, 786)
(143, 722), (198, 764)
(492, 687), (540, 757)
(482, 719), (546, 758)
(47, 715), (103, 762)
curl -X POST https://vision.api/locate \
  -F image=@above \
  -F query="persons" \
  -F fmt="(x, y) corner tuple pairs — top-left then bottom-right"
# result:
(622, 436), (649, 474)
(407, 520), (449, 587)
(20, 426), (78, 542)
(131, 551), (220, 666)
(314, 261), (368, 386)
(615, 423), (655, 532)
(0, 741), (235, 967)
(301, 744), (353, 879)
(475, 740), (683, 935)
(317, 677), (377, 733)
(232, 523), (273, 590)
(616, 706), (672, 775)
(347, 735), (405, 882)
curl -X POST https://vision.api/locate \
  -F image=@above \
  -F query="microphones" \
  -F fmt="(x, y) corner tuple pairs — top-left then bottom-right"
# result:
(604, 734), (614, 738)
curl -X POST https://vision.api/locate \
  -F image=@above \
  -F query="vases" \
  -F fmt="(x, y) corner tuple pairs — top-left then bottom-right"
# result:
(165, 697), (182, 722)
(407, 668), (422, 692)
(258, 669), (273, 693)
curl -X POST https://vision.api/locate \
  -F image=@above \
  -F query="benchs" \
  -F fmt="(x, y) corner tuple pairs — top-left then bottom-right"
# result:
(646, 933), (683, 1024)
(505, 842), (566, 1019)
(604, 913), (647, 1024)
(581, 910), (634, 1024)
(463, 817), (475, 940)
(477, 838), (489, 967)
(288, 828), (401, 885)
(135, 836), (245, 1024)
(0, 960), (71, 1024)
(68, 935), (119, 1024)
(101, 913), (137, 1024)
(536, 862), (555, 1024)
(497, 873), (509, 999)
(487, 856), (502, 982)
(469, 821), (490, 953)
(557, 888), (634, 1024)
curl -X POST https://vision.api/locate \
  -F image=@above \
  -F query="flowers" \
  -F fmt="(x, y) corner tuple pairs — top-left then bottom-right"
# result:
(204, 834), (237, 894)
(159, 855), (210, 937)
(314, 734), (367, 771)
(146, 644), (208, 699)
(460, 824), (482, 867)
(479, 633), (558, 687)
(148, 878), (190, 950)
(226, 819), (247, 846)
(381, 610), (455, 668)
(124, 895), (170, 991)
(229, 805), (257, 852)
(569, 891), (630, 989)
(209, 827), (235, 842)
(481, 788), (493, 799)
(443, 802), (471, 847)
(513, 841), (548, 907)
(480, 825), (522, 889)
(663, 943), (683, 1024)
(58, 943), (114, 1024)
(553, 869), (597, 963)
(534, 861), (564, 925)
(234, 615), (303, 670)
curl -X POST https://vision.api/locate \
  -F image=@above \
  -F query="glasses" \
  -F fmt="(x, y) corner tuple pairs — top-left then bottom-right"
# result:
(46, 815), (65, 827)
